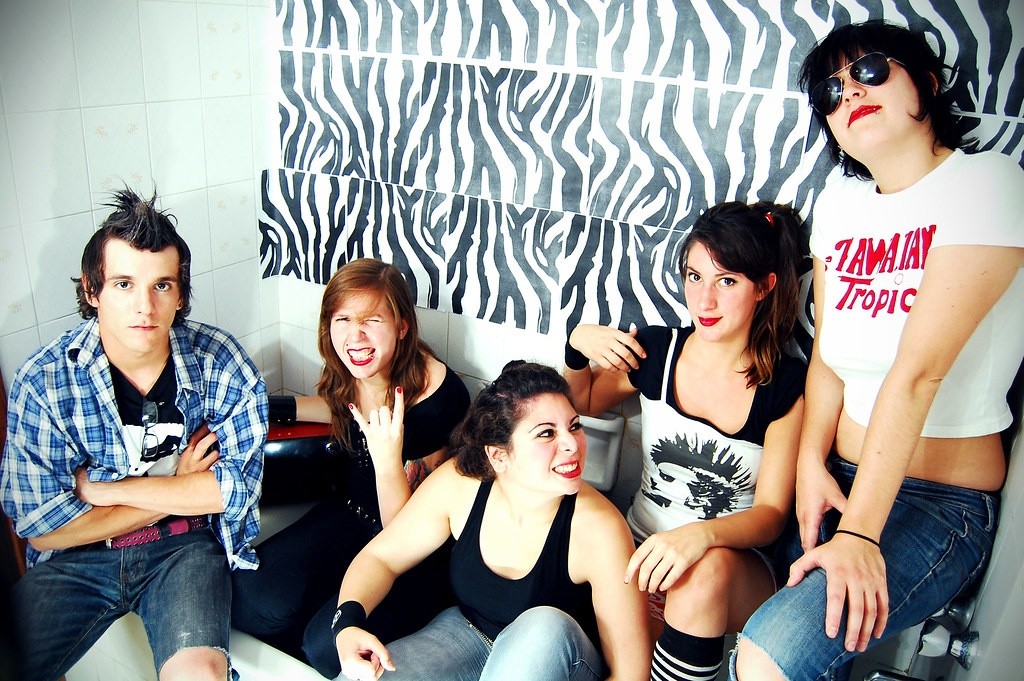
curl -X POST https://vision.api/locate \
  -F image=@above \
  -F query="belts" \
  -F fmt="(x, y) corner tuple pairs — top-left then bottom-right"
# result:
(344, 498), (379, 530)
(104, 514), (209, 551)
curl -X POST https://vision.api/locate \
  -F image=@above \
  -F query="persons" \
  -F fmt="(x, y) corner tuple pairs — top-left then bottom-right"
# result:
(730, 19), (1024, 681)
(0, 180), (269, 681)
(563, 202), (803, 681)
(231, 259), (470, 680)
(330, 360), (652, 681)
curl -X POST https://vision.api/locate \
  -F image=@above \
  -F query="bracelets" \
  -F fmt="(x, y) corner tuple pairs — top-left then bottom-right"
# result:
(267, 395), (297, 425)
(331, 600), (366, 643)
(565, 337), (589, 371)
(836, 529), (880, 547)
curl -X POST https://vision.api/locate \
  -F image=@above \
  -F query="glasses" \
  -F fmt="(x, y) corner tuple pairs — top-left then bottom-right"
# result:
(806, 51), (906, 116)
(141, 397), (160, 462)
